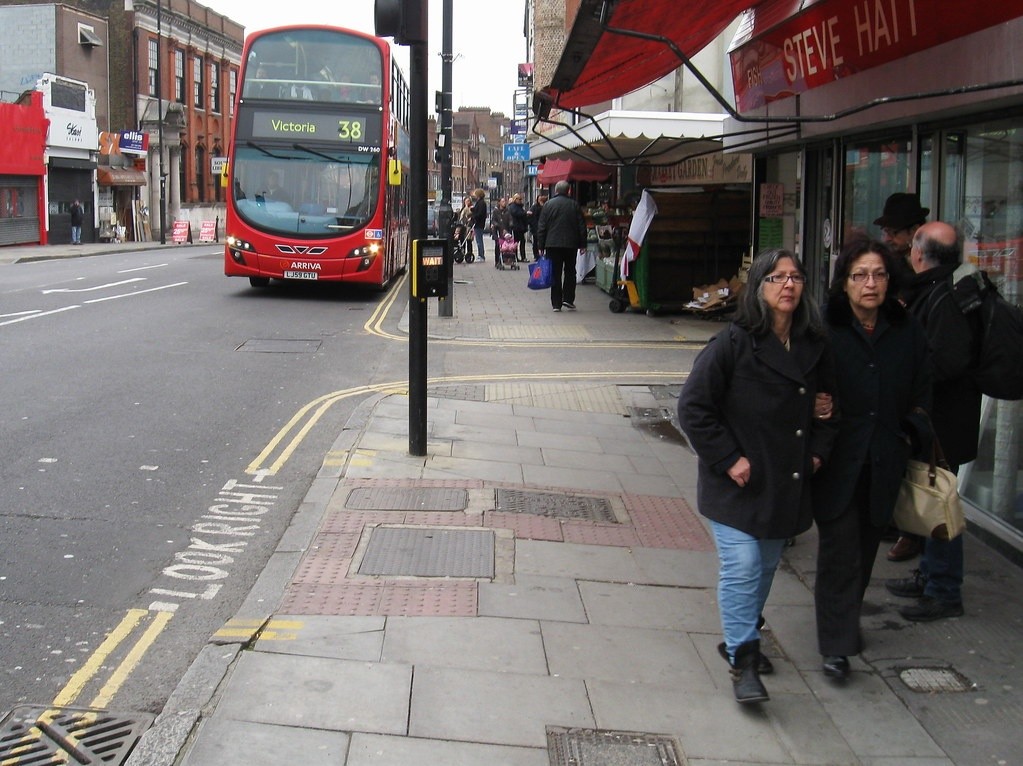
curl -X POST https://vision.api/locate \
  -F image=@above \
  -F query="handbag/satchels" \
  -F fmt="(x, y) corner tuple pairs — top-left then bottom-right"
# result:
(526, 250), (553, 290)
(894, 408), (967, 544)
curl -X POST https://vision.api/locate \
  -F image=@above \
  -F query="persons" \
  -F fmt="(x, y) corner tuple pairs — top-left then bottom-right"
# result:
(529, 192), (549, 262)
(246, 66), (279, 98)
(358, 71), (380, 102)
(490, 192), (530, 267)
(256, 171), (289, 202)
(70, 199), (83, 245)
(799, 238), (922, 688)
(536, 180), (587, 311)
(677, 248), (836, 703)
(330, 73), (358, 101)
(872, 190), (982, 622)
(452, 188), (487, 262)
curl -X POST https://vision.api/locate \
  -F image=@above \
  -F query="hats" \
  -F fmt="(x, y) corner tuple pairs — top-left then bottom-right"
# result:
(512, 193), (520, 203)
(508, 198), (513, 205)
(873, 192), (930, 229)
(503, 233), (512, 239)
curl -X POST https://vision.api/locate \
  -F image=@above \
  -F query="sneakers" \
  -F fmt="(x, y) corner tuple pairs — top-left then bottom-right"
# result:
(885, 568), (927, 597)
(897, 593), (964, 621)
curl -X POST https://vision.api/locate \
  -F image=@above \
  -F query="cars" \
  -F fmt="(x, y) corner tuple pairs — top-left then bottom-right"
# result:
(427, 209), (439, 238)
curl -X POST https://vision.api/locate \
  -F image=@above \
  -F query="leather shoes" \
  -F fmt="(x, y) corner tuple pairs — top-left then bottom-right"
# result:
(887, 536), (923, 562)
(822, 654), (849, 677)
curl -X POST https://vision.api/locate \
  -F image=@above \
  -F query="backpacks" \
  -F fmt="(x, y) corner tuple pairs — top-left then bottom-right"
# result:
(922, 262), (1023, 400)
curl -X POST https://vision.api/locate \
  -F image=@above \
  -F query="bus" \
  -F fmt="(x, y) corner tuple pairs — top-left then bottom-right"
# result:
(451, 191), (471, 213)
(220, 24), (410, 296)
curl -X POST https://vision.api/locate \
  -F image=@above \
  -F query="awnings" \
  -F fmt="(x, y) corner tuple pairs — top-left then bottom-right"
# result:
(540, 158), (610, 183)
(531, 0), (1023, 167)
(97, 165), (147, 186)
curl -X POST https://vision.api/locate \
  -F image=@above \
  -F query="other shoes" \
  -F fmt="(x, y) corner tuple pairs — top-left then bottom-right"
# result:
(552, 307), (561, 312)
(474, 256), (485, 263)
(562, 300), (576, 308)
(514, 258), (530, 262)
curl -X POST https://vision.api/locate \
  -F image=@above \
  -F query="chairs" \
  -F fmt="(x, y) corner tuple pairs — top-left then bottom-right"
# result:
(341, 183), (365, 215)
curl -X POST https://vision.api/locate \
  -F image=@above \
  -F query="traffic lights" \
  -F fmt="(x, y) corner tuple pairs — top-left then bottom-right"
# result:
(374, 0), (421, 46)
(435, 139), (442, 163)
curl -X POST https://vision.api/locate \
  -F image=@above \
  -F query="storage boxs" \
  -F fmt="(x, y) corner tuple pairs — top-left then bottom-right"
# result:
(595, 225), (613, 243)
(100, 237), (111, 243)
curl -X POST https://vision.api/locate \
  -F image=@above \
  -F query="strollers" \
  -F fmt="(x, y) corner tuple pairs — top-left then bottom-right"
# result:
(452, 220), (476, 264)
(496, 229), (520, 271)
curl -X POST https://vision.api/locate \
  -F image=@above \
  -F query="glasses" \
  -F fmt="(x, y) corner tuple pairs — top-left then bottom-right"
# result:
(517, 198), (522, 201)
(879, 226), (906, 238)
(764, 274), (807, 284)
(848, 271), (889, 283)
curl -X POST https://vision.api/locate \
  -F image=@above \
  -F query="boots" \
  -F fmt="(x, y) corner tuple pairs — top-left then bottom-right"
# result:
(755, 614), (773, 674)
(717, 639), (770, 704)
